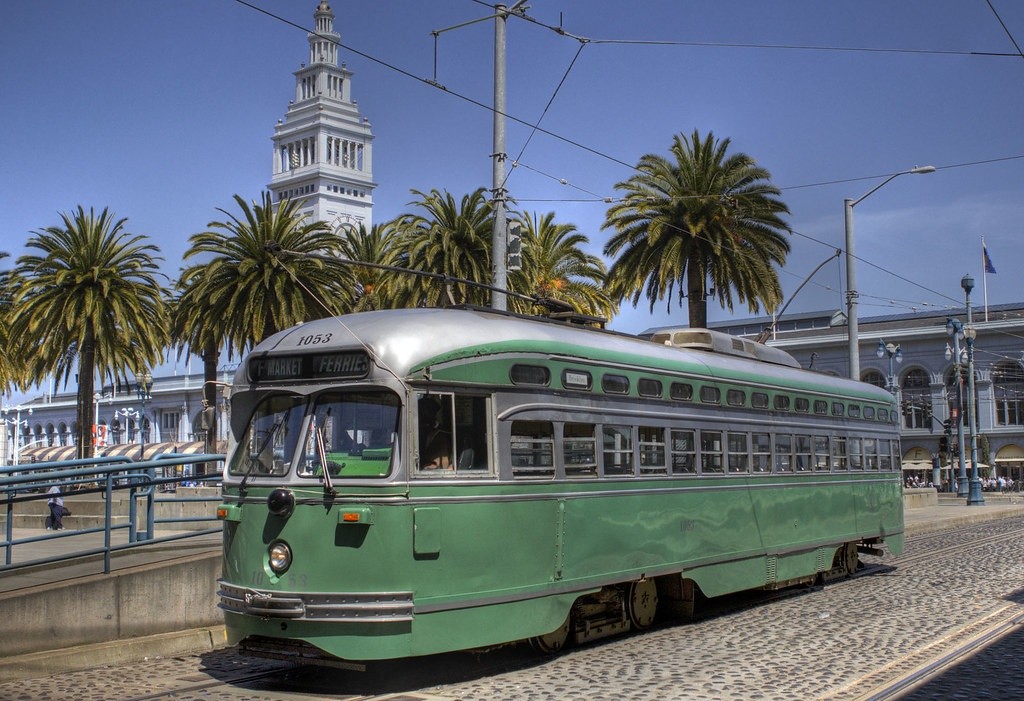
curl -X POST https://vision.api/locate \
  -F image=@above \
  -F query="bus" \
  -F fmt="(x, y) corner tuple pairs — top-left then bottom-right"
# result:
(199, 295), (906, 682)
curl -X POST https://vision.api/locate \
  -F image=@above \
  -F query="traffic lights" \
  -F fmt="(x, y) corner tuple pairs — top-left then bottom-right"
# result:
(939, 437), (948, 453)
(925, 401), (933, 419)
(943, 418), (951, 434)
(902, 401), (906, 416)
(920, 401), (926, 419)
(907, 400), (914, 416)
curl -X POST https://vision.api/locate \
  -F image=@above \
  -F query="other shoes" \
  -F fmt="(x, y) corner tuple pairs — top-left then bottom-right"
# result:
(47, 527), (52, 531)
(57, 527), (65, 531)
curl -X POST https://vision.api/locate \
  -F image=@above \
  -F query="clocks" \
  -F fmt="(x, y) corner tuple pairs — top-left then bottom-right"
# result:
(328, 216), (361, 259)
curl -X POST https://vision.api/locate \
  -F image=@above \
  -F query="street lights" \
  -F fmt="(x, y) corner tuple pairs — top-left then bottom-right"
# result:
(135, 369), (153, 492)
(94, 393), (105, 485)
(115, 407), (139, 446)
(960, 273), (987, 506)
(875, 338), (903, 397)
(3, 403), (33, 476)
(842, 166), (938, 382)
(943, 316), (969, 498)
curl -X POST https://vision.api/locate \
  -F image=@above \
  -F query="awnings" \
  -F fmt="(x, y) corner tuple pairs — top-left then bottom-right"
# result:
(18, 441), (229, 489)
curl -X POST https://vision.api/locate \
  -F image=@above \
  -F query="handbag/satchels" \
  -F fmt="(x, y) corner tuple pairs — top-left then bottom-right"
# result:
(62, 506), (71, 516)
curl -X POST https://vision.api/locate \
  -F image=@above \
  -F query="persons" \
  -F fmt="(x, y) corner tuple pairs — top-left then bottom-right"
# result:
(46, 479), (65, 532)
(416, 397), (450, 471)
(904, 472), (1019, 492)
